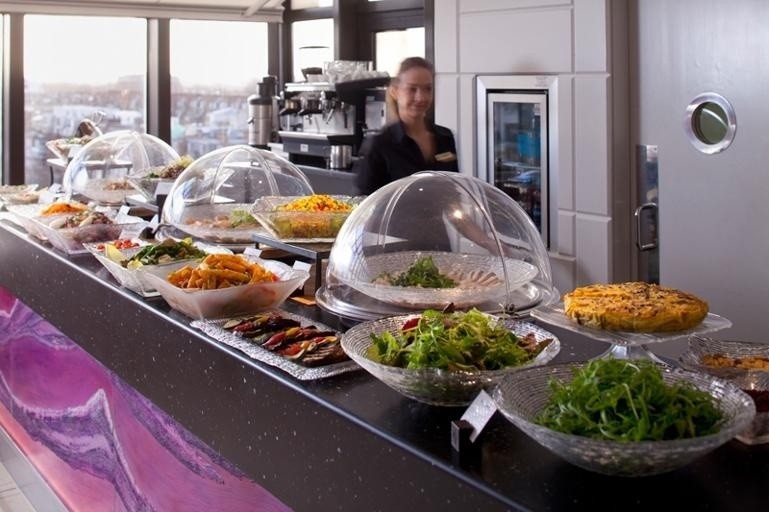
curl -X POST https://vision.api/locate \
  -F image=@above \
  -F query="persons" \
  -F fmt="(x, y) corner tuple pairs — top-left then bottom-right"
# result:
(352, 57), (510, 257)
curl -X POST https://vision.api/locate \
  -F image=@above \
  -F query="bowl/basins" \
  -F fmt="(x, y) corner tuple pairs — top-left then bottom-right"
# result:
(338, 310), (564, 409)
(326, 246), (540, 312)
(1, 183), (312, 324)
(492, 360), (755, 474)
(646, 323), (769, 394)
(44, 138), (81, 164)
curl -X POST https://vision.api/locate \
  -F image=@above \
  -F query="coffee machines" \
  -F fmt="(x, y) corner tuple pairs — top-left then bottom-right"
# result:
(276, 73), (396, 173)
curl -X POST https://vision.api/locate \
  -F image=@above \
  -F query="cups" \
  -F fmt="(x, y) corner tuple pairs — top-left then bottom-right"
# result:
(302, 60), (376, 83)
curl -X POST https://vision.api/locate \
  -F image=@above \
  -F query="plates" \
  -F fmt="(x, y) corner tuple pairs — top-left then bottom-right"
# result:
(248, 193), (368, 244)
(189, 307), (383, 382)
(536, 305), (734, 347)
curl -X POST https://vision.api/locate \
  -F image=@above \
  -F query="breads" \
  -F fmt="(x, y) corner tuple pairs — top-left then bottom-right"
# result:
(564, 281), (709, 333)
